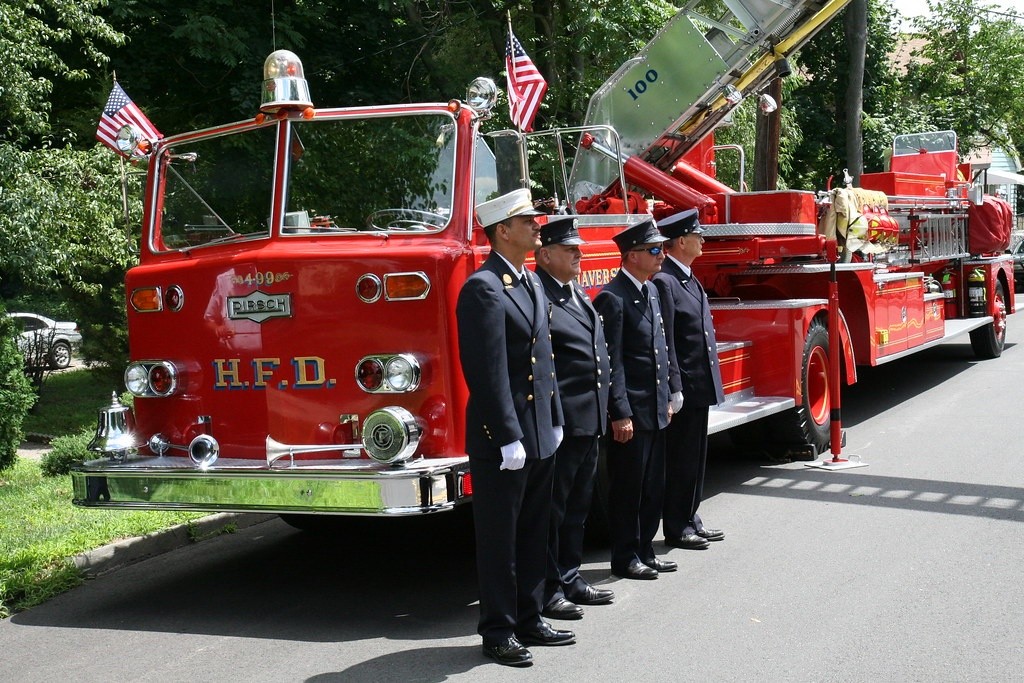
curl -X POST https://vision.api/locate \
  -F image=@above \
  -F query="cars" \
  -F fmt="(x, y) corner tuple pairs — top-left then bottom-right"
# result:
(1002, 231), (1024, 284)
(0, 310), (83, 368)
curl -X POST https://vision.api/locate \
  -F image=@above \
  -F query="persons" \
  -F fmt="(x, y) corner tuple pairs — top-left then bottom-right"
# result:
(532, 214), (614, 619)
(455, 187), (575, 665)
(593, 214), (677, 579)
(651, 208), (725, 549)
(204, 259), (262, 348)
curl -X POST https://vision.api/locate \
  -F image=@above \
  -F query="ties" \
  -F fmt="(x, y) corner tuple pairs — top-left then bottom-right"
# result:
(562, 285), (572, 299)
(641, 284), (649, 303)
(690, 271), (696, 283)
(520, 274), (534, 303)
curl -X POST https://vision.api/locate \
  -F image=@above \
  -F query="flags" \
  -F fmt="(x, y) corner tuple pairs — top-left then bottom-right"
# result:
(96, 80), (171, 170)
(506, 28), (547, 132)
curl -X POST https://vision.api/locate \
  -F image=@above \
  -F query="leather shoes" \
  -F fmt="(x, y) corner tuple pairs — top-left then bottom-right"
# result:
(610, 560), (658, 581)
(694, 526), (725, 541)
(540, 598), (584, 620)
(482, 635), (533, 665)
(516, 621), (576, 648)
(641, 556), (678, 572)
(665, 532), (710, 548)
(565, 584), (614, 604)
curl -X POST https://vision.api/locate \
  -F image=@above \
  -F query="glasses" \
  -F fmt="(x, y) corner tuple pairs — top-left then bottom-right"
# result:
(630, 244), (662, 255)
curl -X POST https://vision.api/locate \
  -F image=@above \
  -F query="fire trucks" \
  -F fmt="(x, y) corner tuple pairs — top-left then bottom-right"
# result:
(66, 0), (1015, 551)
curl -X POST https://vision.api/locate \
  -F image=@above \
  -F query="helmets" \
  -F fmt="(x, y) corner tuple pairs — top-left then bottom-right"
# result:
(862, 204), (884, 242)
(881, 208), (899, 242)
(872, 205), (894, 242)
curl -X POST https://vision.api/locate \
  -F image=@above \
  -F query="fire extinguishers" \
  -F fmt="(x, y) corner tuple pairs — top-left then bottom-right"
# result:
(942, 268), (959, 319)
(967, 265), (986, 317)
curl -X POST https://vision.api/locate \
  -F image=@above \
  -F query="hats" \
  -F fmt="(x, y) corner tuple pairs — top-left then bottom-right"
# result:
(539, 217), (587, 247)
(612, 217), (670, 254)
(475, 188), (547, 227)
(657, 208), (706, 240)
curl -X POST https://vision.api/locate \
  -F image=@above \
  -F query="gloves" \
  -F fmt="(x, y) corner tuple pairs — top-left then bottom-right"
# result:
(666, 391), (684, 413)
(551, 425), (563, 450)
(500, 440), (526, 470)
